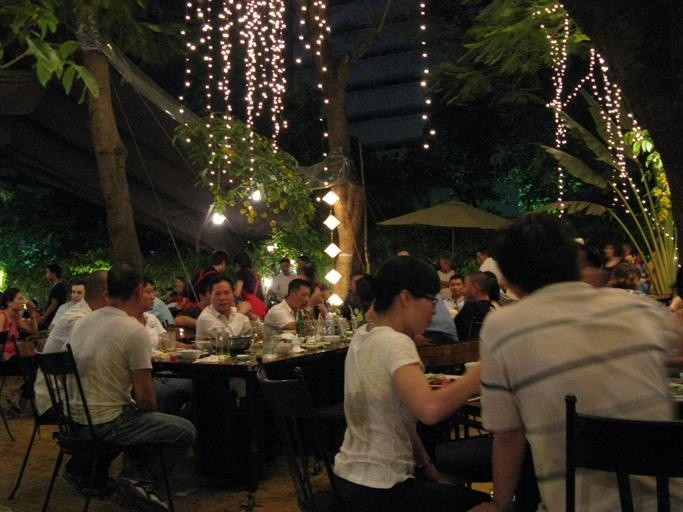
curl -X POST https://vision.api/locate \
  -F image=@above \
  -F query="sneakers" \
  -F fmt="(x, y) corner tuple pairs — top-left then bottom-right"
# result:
(63, 463), (99, 499)
(117, 478), (167, 509)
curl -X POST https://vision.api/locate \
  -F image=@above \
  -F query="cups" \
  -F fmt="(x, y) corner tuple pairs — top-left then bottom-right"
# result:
(326, 320), (335, 336)
(215, 332), (229, 362)
(338, 317), (347, 339)
(164, 332), (176, 352)
(464, 361), (479, 372)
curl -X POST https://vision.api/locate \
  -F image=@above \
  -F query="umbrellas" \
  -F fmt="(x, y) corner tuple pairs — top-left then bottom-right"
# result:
(376, 199), (513, 252)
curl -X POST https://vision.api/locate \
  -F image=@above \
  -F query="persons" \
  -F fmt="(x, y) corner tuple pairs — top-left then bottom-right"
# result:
(0, 287), (43, 418)
(434, 256), (456, 280)
(297, 256), (314, 280)
(40, 269), (110, 501)
(300, 283), (321, 326)
(668, 263), (683, 311)
(264, 278), (310, 335)
(22, 297), (41, 322)
(196, 250), (229, 307)
(453, 271), (494, 342)
(269, 259), (295, 301)
(70, 263), (197, 512)
(595, 240), (629, 288)
(172, 276), (188, 308)
(441, 272), (466, 315)
(49, 279), (85, 332)
(480, 211), (683, 511)
(197, 275), (254, 488)
(175, 279), (251, 329)
(131, 271), (194, 482)
(318, 282), (335, 316)
(330, 254), (492, 512)
(8, 263), (67, 414)
(232, 250), (267, 320)
(476, 250), (505, 287)
(340, 271), (372, 318)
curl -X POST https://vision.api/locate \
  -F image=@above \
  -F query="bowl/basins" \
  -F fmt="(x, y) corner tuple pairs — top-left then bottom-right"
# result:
(323, 335), (341, 343)
(181, 350), (201, 360)
(277, 343), (292, 354)
(196, 341), (210, 349)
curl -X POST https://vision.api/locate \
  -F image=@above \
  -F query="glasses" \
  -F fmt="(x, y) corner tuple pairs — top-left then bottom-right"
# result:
(425, 296), (440, 307)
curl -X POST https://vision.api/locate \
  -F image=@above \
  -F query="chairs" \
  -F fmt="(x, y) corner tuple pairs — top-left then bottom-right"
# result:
(256, 357), (335, 512)
(563, 394), (682, 511)
(8, 336), (58, 502)
(34, 343), (172, 512)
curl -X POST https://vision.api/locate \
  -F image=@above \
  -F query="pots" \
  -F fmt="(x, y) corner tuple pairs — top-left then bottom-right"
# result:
(161, 320), (195, 342)
(206, 334), (258, 355)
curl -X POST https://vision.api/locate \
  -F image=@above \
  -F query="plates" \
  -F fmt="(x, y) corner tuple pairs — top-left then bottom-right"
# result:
(425, 373), (462, 389)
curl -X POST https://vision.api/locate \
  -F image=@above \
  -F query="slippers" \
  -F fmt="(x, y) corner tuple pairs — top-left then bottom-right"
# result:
(7, 398), (32, 418)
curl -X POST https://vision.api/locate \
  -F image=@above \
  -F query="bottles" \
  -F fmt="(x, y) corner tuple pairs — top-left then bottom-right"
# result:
(295, 308), (306, 336)
